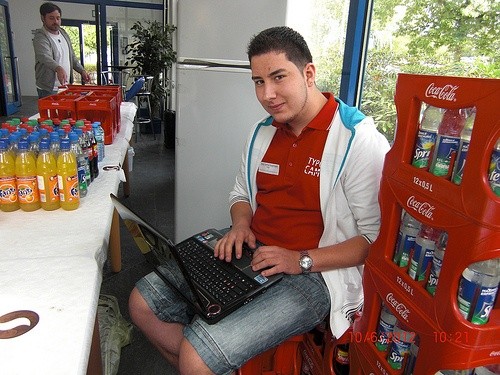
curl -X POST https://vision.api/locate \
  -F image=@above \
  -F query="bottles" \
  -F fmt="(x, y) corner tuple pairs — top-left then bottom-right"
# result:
(377, 211), (500, 374)
(0, 116), (105, 211)
(412, 102), (500, 195)
(334, 312), (360, 375)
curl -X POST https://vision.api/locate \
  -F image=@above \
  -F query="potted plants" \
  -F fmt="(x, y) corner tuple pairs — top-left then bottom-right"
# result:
(121, 17), (177, 133)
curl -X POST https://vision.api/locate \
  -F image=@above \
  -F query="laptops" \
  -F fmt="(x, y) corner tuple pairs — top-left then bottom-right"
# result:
(109, 193), (283, 324)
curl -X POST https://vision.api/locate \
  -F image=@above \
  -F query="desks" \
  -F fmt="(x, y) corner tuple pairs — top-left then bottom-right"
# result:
(0, 103), (137, 375)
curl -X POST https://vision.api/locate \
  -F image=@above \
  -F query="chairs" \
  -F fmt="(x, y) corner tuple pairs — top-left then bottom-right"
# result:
(101, 70), (154, 144)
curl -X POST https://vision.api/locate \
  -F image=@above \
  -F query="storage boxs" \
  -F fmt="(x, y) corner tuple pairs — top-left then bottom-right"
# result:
(38, 85), (121, 145)
(237, 74), (500, 375)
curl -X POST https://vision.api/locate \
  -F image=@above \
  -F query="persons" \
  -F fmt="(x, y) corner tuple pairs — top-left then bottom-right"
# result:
(34, 2), (91, 99)
(129, 26), (391, 375)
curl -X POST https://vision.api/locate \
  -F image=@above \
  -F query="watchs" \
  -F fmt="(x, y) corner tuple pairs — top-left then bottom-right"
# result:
(298, 250), (313, 276)
